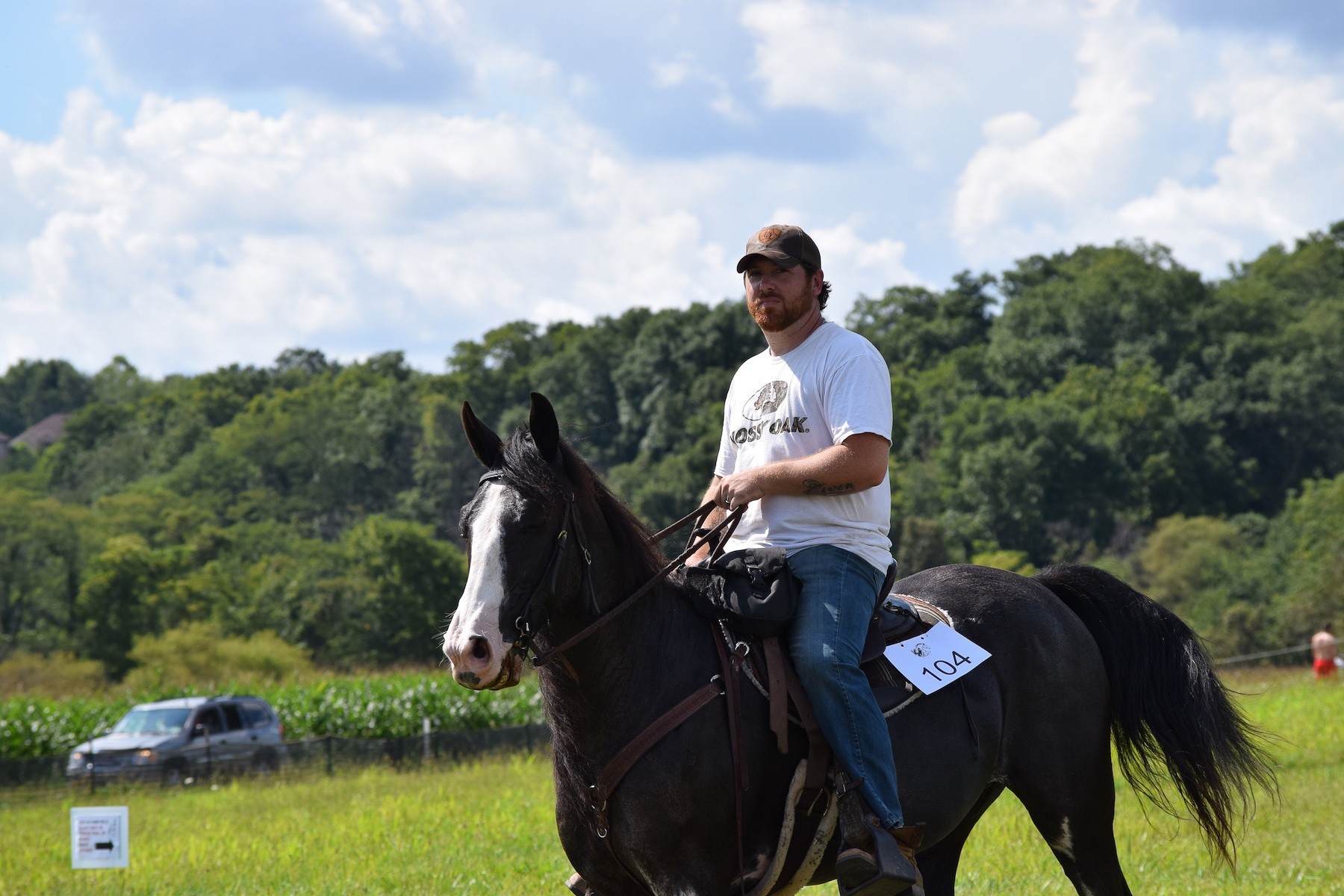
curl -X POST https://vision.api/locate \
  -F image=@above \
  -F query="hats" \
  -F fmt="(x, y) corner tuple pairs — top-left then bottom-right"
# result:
(737, 225), (821, 273)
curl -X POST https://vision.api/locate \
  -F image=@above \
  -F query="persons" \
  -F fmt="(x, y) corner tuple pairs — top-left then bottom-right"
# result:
(1311, 620), (1337, 678)
(682, 224), (923, 895)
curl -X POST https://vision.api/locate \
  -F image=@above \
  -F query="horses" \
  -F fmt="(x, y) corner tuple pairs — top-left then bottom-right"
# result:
(441, 381), (1298, 896)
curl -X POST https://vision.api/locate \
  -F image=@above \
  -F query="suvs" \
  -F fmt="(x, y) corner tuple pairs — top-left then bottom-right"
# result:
(65, 693), (289, 788)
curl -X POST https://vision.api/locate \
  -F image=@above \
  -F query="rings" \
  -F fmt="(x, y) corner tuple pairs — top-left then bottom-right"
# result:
(725, 500), (730, 504)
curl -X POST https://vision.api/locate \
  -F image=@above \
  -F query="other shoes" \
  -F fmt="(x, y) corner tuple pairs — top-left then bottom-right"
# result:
(836, 843), (928, 896)
